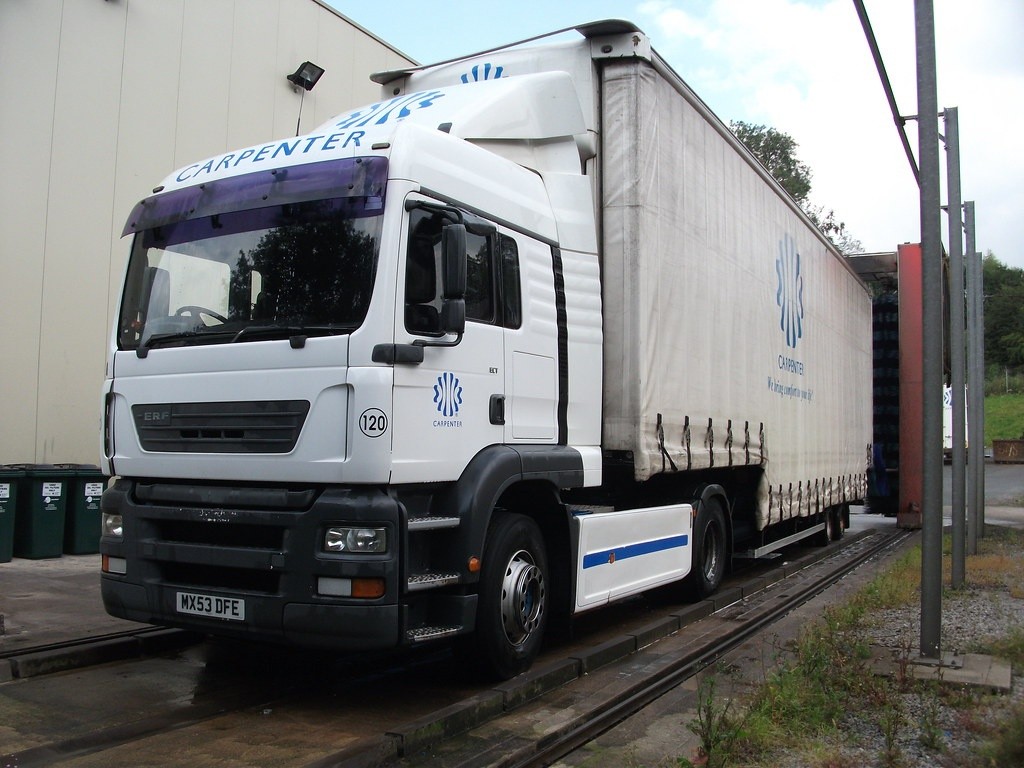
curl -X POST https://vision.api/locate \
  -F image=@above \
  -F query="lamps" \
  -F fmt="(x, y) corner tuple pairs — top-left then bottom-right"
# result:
(287, 61), (325, 91)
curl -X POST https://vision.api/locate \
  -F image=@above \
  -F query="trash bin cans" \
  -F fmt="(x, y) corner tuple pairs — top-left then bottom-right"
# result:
(0, 463), (111, 564)
(993, 439), (1024, 463)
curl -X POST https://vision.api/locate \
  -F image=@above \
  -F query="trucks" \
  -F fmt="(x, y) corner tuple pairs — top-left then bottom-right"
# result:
(96, 16), (878, 691)
